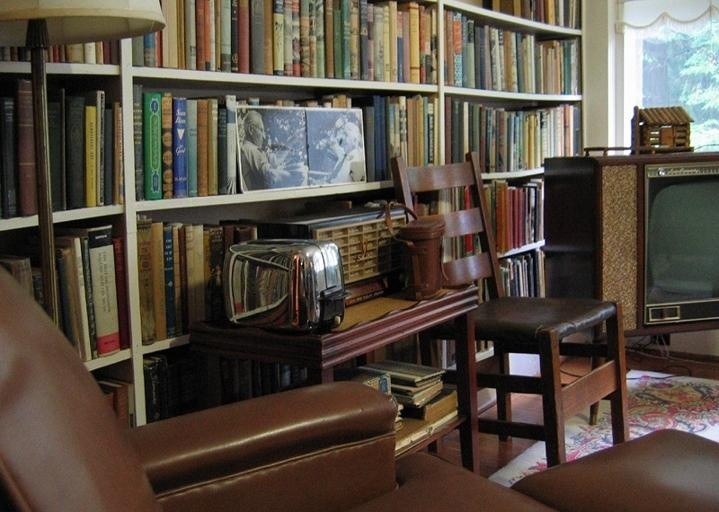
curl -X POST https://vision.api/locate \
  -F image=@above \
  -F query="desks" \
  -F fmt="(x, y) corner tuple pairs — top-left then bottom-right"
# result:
(190, 288), (480, 475)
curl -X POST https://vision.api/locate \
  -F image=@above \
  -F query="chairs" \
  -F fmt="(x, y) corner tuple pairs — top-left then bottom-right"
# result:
(390, 152), (630, 469)
(0, 264), (559, 512)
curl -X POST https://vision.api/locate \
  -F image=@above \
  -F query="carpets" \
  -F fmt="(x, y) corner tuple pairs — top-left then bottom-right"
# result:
(487, 368), (719, 488)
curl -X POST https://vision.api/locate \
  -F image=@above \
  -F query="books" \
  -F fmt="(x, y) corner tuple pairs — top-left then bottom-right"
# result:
(2, 3), (581, 436)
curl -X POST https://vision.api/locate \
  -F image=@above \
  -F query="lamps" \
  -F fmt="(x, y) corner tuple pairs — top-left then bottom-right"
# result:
(0, 0), (164, 329)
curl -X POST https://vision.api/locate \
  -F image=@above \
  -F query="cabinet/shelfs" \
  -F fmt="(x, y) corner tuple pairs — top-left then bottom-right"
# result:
(0, 0), (587, 428)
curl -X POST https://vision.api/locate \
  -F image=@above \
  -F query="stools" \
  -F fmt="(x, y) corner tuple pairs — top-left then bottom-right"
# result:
(511, 428), (719, 512)
